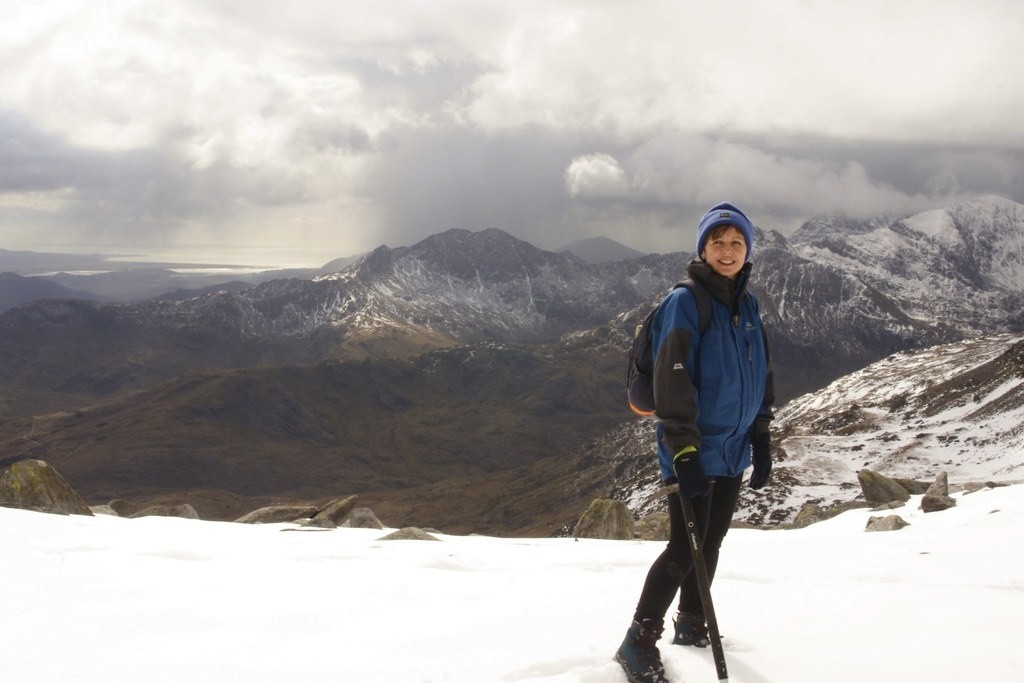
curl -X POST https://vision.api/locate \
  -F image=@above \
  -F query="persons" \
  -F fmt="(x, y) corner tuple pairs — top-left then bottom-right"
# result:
(612, 202), (774, 682)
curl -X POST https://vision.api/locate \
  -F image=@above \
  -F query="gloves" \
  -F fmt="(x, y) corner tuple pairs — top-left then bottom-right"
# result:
(672, 445), (709, 500)
(749, 432), (771, 489)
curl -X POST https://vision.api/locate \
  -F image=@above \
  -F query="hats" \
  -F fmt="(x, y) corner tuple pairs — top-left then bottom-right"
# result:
(696, 201), (754, 261)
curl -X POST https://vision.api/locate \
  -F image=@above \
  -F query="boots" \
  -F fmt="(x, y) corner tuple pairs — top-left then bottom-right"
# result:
(671, 611), (710, 647)
(616, 613), (668, 683)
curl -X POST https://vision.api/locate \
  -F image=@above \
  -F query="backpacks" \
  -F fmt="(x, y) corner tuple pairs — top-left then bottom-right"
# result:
(627, 278), (712, 415)
